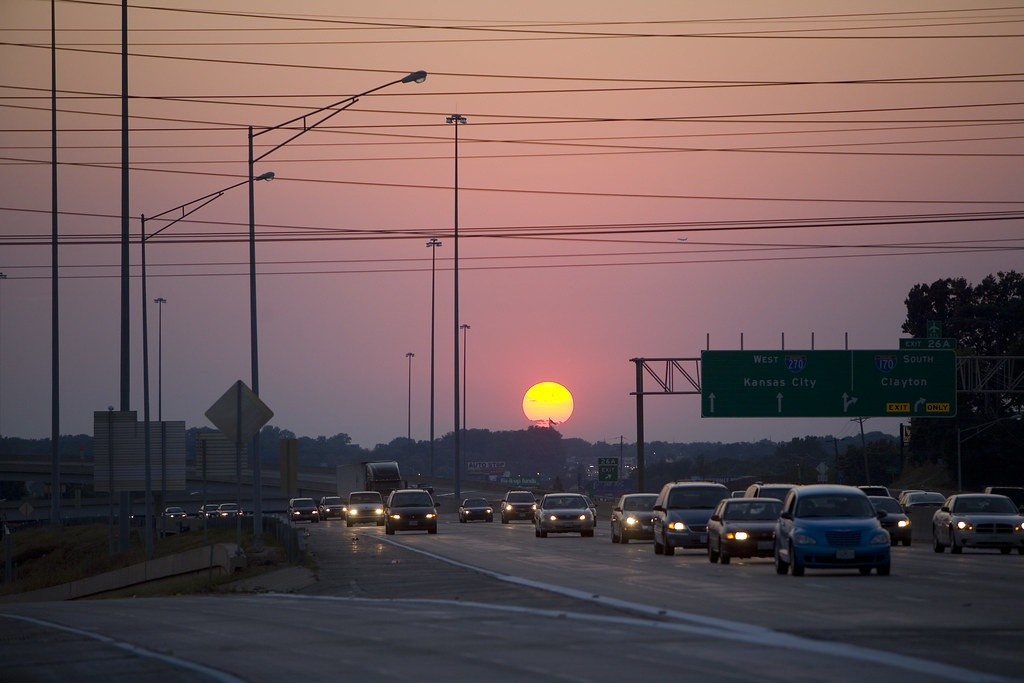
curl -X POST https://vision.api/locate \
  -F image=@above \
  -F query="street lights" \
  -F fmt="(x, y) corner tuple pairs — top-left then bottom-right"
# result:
(445, 112), (468, 501)
(459, 323), (471, 461)
(251, 68), (430, 542)
(154, 298), (167, 516)
(143, 172), (276, 555)
(404, 352), (415, 454)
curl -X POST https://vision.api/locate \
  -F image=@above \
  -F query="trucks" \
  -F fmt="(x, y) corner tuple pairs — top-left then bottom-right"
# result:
(337, 459), (403, 499)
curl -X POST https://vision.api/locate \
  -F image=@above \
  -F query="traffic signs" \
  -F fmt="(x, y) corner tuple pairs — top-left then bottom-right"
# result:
(700, 348), (958, 419)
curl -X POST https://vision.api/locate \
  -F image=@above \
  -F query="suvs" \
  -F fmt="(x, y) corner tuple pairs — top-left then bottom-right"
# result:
(318, 496), (347, 521)
(342, 490), (387, 527)
(383, 487), (440, 536)
(652, 480), (734, 556)
(980, 486), (1024, 516)
(500, 490), (541, 525)
(728, 481), (892, 518)
(286, 497), (321, 523)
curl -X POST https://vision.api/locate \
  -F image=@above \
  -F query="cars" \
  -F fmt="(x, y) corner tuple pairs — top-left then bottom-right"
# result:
(458, 497), (494, 523)
(864, 496), (913, 547)
(895, 490), (948, 519)
(774, 484), (890, 578)
(162, 506), (188, 519)
(706, 498), (785, 564)
(610, 493), (660, 545)
(198, 503), (220, 520)
(216, 502), (246, 519)
(932, 493), (1024, 555)
(533, 492), (598, 538)
